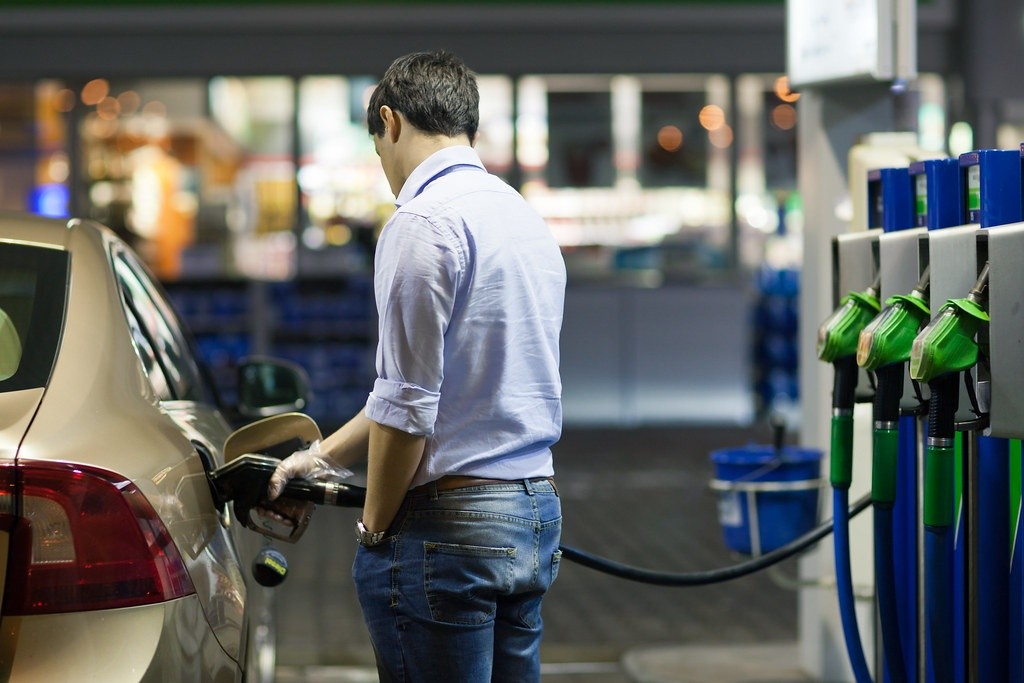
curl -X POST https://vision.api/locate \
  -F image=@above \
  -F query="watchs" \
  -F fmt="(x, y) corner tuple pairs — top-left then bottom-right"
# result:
(353, 518), (384, 545)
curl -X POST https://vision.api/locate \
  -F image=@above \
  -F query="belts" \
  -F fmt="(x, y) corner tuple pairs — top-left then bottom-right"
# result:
(437, 474), (554, 489)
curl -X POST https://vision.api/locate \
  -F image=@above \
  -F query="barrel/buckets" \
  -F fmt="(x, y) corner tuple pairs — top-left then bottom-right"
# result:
(710, 448), (822, 553)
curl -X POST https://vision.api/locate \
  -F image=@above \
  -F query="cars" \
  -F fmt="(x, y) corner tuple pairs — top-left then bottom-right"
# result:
(1, 203), (322, 683)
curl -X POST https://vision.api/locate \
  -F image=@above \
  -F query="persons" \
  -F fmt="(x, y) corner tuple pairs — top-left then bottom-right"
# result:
(258, 51), (566, 682)
